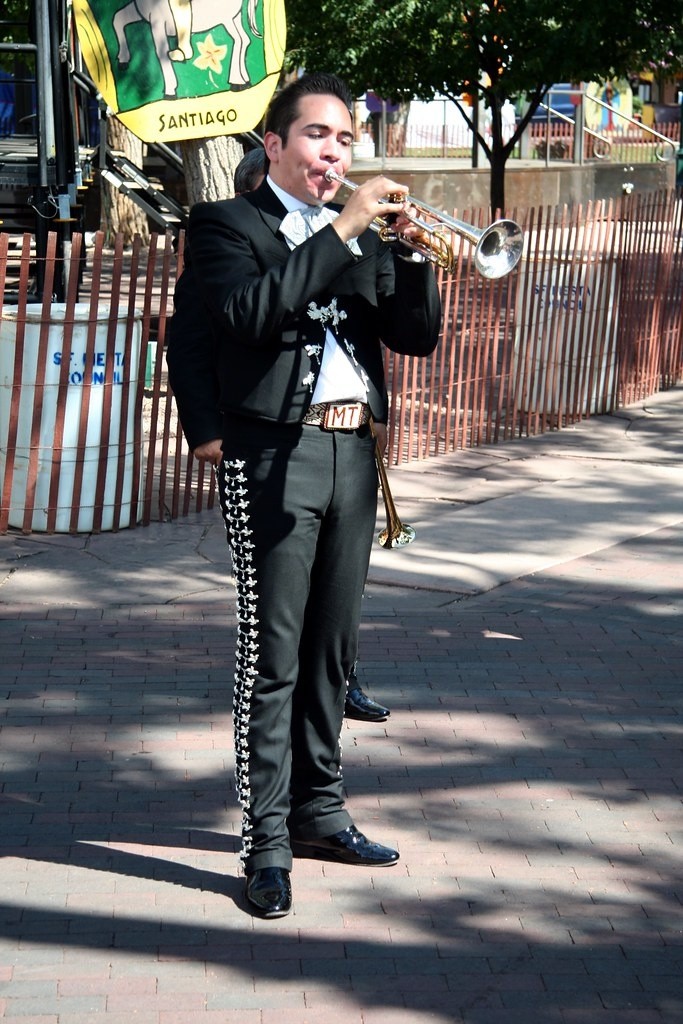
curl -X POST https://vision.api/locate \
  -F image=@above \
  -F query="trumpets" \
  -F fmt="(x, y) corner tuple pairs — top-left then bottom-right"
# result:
(371, 427), (417, 550)
(325, 168), (525, 280)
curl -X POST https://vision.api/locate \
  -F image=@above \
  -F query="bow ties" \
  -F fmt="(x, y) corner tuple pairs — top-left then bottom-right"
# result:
(278, 206), (363, 256)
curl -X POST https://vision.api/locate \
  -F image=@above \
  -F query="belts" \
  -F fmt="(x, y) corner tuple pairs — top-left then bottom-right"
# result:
(305, 400), (377, 434)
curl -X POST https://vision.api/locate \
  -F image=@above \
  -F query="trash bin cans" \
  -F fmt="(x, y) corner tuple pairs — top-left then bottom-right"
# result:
(0, 302), (145, 531)
(515, 251), (621, 415)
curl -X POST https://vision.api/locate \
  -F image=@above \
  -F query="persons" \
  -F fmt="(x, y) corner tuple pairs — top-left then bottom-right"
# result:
(166, 75), (442, 919)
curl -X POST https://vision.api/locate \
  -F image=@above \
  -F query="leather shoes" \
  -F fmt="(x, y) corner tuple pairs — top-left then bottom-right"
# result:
(244, 867), (292, 918)
(345, 689), (390, 722)
(289, 824), (400, 867)
